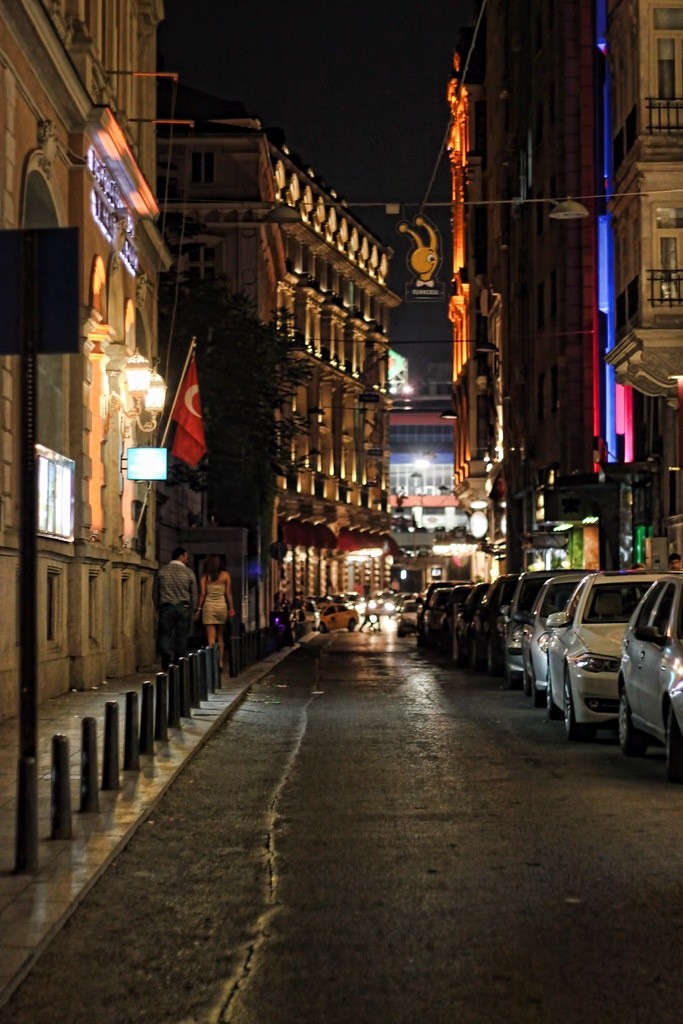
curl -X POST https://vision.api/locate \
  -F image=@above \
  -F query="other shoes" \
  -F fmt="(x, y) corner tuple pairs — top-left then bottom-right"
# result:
(219, 667), (223, 674)
(161, 657), (171, 672)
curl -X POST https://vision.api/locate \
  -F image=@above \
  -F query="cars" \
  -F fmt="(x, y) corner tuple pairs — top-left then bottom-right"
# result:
(393, 567), (683, 782)
(294, 589), (396, 634)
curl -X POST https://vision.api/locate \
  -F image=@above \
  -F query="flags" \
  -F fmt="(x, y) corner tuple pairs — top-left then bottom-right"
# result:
(171, 357), (207, 467)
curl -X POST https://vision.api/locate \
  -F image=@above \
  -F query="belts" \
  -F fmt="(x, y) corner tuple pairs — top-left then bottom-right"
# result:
(162, 601), (190, 606)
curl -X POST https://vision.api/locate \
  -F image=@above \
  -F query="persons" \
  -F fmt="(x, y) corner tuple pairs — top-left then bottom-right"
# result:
(669, 553), (683, 571)
(358, 606), (381, 631)
(196, 556), (235, 671)
(295, 591), (306, 608)
(274, 591), (290, 611)
(157, 547), (198, 671)
(298, 607), (306, 621)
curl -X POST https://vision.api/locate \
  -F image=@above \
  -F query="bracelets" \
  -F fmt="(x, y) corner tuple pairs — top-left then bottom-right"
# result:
(197, 607), (201, 610)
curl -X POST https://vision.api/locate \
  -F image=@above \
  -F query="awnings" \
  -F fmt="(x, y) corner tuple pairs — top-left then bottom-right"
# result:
(338, 527), (399, 555)
(283, 519), (337, 548)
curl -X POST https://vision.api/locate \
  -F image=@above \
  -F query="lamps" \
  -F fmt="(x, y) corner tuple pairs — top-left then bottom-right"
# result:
(108, 348), (167, 432)
